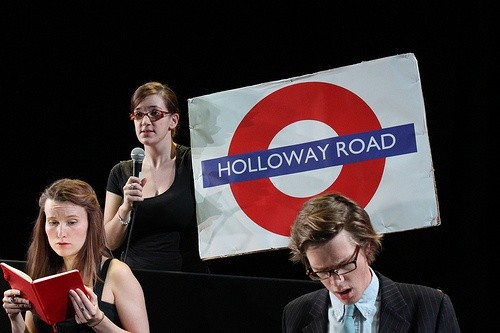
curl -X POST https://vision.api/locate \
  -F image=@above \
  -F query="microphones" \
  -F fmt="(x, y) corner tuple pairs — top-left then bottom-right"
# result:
(131, 146), (145, 204)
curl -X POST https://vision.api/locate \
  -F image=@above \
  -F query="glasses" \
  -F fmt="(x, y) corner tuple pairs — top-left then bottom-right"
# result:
(129, 108), (172, 122)
(304, 245), (360, 281)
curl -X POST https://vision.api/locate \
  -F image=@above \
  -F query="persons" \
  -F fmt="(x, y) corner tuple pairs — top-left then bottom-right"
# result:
(102, 82), (200, 333)
(281, 192), (461, 333)
(2, 179), (150, 333)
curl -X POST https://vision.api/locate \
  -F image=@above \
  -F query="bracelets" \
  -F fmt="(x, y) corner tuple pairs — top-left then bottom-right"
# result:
(116, 211), (131, 225)
(87, 311), (104, 328)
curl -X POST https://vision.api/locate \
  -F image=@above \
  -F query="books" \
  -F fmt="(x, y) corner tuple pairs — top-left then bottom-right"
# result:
(0, 262), (88, 326)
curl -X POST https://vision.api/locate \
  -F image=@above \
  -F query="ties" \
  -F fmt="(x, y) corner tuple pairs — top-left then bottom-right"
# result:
(339, 303), (356, 333)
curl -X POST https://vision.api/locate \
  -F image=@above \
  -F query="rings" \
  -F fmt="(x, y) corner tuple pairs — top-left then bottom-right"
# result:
(80, 307), (85, 312)
(130, 184), (134, 189)
(10, 297), (15, 302)
